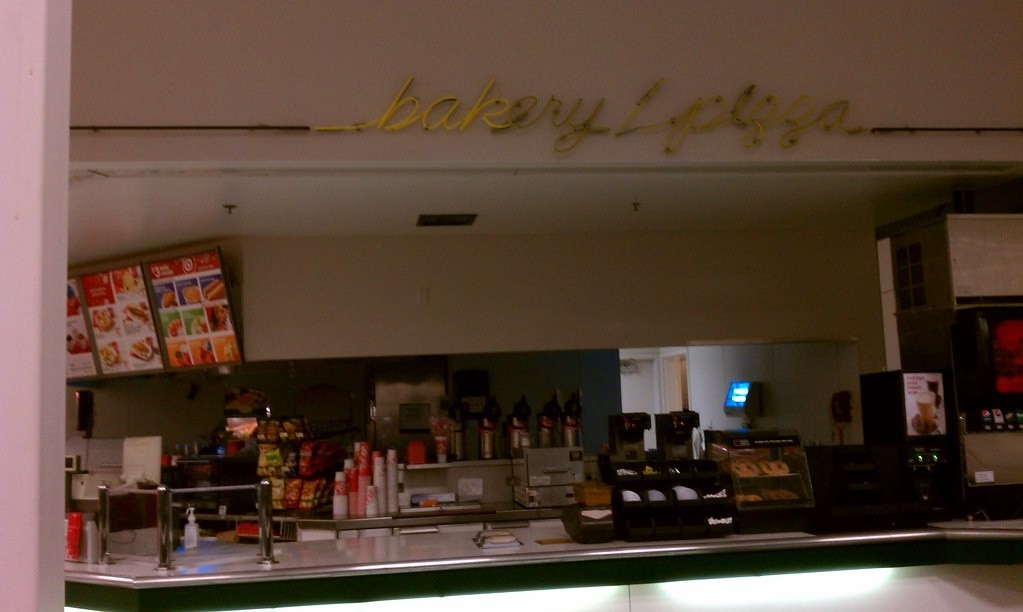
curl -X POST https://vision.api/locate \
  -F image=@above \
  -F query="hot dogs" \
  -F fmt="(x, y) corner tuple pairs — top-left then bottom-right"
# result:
(203, 278), (224, 300)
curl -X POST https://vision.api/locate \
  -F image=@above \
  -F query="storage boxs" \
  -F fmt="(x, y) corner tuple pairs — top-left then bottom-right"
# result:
(570, 482), (612, 507)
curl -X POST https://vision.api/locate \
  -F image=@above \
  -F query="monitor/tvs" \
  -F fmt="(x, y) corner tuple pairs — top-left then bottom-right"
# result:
(123, 436), (162, 484)
(725, 380), (762, 419)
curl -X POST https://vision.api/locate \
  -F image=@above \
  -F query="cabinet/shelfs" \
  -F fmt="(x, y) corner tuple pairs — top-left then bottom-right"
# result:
(704, 428), (819, 535)
